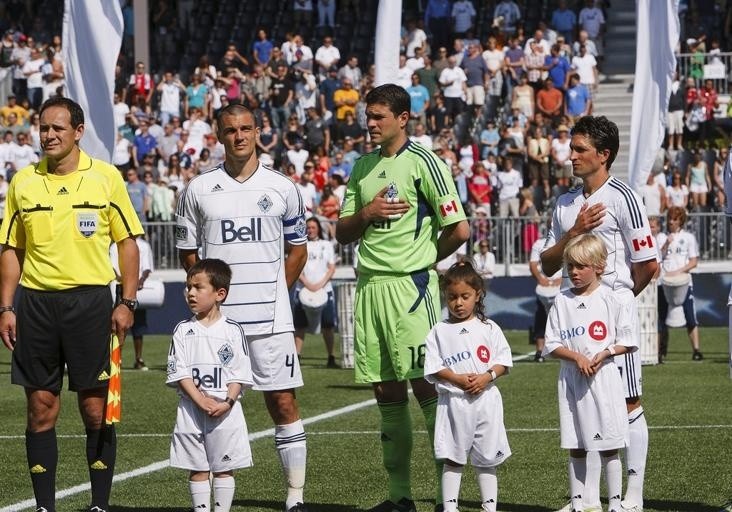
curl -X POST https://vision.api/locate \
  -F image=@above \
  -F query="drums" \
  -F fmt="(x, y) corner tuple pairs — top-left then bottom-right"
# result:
(299, 287), (328, 336)
(111, 277), (165, 308)
(660, 272), (690, 327)
(536, 277), (559, 315)
(336, 282), (360, 369)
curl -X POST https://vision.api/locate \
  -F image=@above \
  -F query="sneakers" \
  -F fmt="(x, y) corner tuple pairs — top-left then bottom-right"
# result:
(327, 360), (341, 369)
(366, 496), (417, 512)
(659, 354), (666, 364)
(692, 349), (703, 361)
(134, 357), (149, 372)
(434, 504), (443, 512)
(534, 351), (545, 362)
(619, 501), (643, 512)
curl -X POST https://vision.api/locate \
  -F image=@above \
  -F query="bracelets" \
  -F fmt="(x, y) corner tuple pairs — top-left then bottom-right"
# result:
(224, 397), (235, 407)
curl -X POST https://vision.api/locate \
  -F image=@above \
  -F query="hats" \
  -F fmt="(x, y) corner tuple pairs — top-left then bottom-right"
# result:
(556, 124), (568, 133)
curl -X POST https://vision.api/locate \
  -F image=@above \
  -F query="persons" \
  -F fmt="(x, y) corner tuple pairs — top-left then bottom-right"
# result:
(641, 1), (732, 367)
(336, 80), (473, 512)
(425, 260), (515, 512)
(108, 1), (375, 366)
(528, 219), (565, 362)
(400, 1), (606, 276)
(164, 255), (257, 512)
(541, 114), (660, 511)
(1, 1), (68, 216)
(174, 99), (312, 511)
(543, 233), (637, 511)
(1, 97), (149, 511)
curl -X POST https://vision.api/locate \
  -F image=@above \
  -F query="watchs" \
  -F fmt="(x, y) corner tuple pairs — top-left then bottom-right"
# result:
(487, 369), (498, 381)
